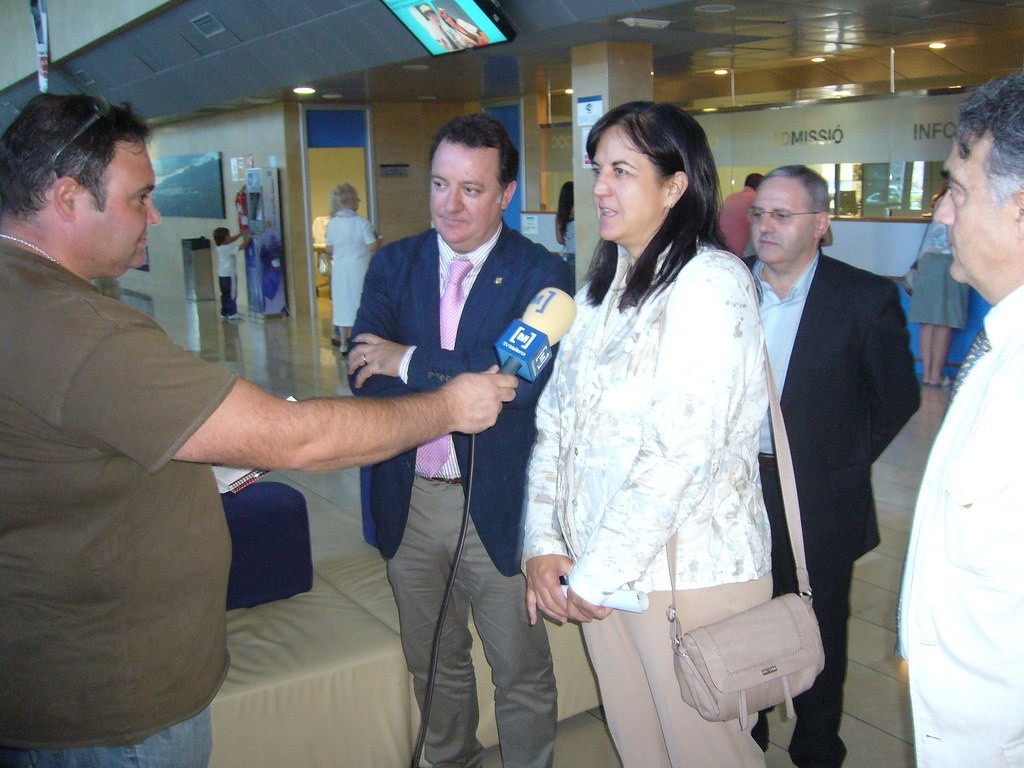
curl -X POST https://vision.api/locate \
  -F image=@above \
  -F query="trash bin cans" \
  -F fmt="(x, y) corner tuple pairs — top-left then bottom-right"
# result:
(182, 237), (216, 302)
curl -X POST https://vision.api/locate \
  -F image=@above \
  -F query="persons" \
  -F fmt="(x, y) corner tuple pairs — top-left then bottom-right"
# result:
(324, 183), (382, 355)
(895, 68), (1024, 768)
(521, 99), (774, 768)
(420, 5), (488, 50)
(555, 182), (574, 276)
(0, 94), (518, 768)
(908, 193), (969, 386)
(715, 173), (766, 259)
(213, 227), (251, 320)
(348, 113), (571, 768)
(734, 165), (923, 768)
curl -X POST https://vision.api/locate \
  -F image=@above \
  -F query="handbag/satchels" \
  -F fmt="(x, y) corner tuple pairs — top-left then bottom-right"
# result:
(898, 259), (918, 298)
(674, 595), (825, 732)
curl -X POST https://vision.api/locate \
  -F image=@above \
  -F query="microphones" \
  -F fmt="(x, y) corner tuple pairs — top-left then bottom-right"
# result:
(493, 286), (578, 382)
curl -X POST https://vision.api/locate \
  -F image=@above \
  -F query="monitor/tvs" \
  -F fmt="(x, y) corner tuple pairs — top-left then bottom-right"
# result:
(382, 1), (517, 58)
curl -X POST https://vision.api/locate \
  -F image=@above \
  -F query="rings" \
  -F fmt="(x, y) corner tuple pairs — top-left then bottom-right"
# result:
(362, 355), (369, 365)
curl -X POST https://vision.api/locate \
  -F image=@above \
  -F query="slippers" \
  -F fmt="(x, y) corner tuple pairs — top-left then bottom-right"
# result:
(921, 374), (955, 388)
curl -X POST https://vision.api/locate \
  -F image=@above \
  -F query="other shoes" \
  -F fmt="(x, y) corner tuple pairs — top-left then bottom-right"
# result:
(338, 345), (348, 356)
(330, 334), (341, 345)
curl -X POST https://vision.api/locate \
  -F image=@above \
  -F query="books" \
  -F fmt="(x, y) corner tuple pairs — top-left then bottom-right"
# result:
(212, 395), (298, 493)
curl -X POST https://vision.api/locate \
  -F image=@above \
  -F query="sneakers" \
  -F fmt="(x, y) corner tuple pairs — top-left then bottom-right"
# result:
(220, 312), (242, 320)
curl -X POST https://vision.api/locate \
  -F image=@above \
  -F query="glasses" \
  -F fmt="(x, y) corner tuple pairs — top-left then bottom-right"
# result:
(34, 93), (110, 185)
(744, 208), (821, 221)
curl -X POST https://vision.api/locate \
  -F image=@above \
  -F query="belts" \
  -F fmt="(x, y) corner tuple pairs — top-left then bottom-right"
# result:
(415, 474), (463, 485)
(757, 452), (779, 469)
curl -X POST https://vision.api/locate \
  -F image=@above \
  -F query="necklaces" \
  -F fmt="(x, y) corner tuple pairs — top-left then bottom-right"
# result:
(0, 233), (59, 264)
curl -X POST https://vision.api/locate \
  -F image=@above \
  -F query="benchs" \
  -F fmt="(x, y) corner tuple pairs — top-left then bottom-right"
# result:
(203, 463), (603, 768)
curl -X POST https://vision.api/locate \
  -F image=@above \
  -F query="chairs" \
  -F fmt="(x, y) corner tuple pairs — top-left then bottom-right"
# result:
(312, 216), (332, 300)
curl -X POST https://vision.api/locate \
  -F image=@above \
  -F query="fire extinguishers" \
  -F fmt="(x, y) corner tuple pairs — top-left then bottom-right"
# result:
(235, 184), (249, 234)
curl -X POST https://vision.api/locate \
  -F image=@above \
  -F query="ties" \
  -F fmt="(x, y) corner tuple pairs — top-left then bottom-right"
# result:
(418, 257), (476, 479)
